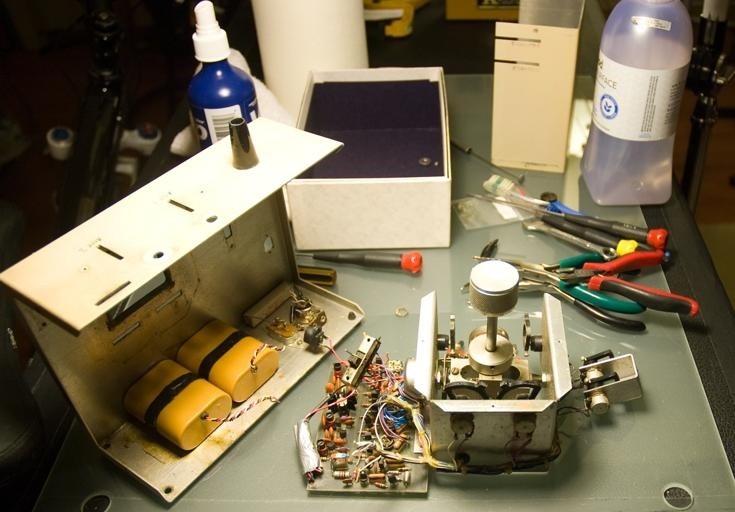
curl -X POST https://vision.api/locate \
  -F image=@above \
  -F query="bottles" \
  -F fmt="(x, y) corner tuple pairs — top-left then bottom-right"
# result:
(578, 0), (693, 207)
(189, 1), (261, 153)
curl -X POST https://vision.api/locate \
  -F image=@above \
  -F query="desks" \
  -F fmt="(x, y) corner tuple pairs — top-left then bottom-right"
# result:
(0, 47), (735, 512)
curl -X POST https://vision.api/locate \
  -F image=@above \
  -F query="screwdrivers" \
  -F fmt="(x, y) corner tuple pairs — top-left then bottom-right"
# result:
(295, 252), (422, 273)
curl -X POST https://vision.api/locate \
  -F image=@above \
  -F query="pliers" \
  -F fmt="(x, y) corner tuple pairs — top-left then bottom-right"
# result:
(472, 251), (699, 331)
